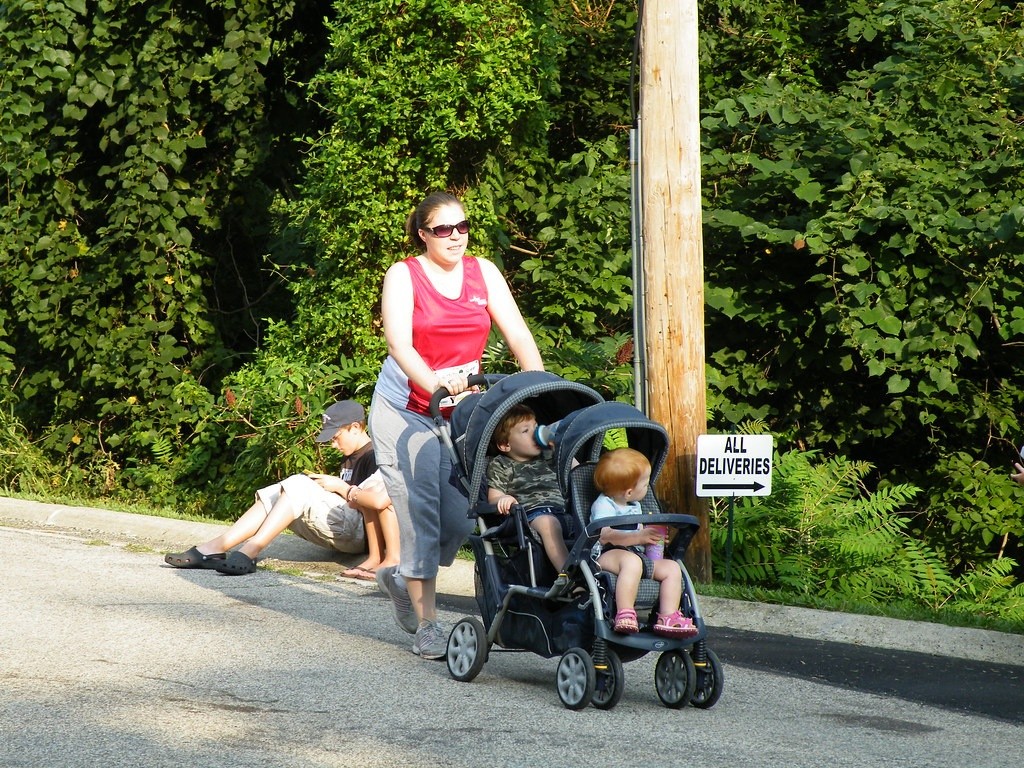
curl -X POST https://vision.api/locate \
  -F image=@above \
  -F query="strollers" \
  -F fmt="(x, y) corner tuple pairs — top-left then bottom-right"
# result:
(428, 368), (725, 711)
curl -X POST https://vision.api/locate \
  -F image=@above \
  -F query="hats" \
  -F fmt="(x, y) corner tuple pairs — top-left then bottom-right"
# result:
(315, 400), (366, 442)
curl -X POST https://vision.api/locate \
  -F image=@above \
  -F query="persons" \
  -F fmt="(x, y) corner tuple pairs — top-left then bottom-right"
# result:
(487, 404), (572, 577)
(165, 399), (380, 581)
(338, 468), (400, 582)
(367, 188), (545, 661)
(591, 449), (701, 638)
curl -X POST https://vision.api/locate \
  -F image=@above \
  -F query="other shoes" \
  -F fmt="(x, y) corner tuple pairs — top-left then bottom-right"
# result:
(568, 584), (587, 599)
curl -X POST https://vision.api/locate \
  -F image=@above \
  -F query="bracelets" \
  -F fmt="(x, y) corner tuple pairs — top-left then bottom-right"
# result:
(347, 485), (361, 503)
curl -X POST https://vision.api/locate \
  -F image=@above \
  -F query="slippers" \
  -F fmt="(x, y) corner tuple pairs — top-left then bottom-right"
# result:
(356, 568), (376, 581)
(340, 564), (367, 578)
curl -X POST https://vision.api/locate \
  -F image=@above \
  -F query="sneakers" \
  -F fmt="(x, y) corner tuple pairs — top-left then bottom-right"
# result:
(376, 565), (418, 637)
(412, 617), (448, 659)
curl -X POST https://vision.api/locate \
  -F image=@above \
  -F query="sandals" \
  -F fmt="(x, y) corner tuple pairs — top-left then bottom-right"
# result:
(615, 608), (638, 630)
(165, 545), (226, 568)
(653, 607), (699, 634)
(216, 551), (256, 574)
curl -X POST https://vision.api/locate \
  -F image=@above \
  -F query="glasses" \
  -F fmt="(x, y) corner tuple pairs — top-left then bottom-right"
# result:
(330, 428), (347, 443)
(421, 220), (471, 238)
